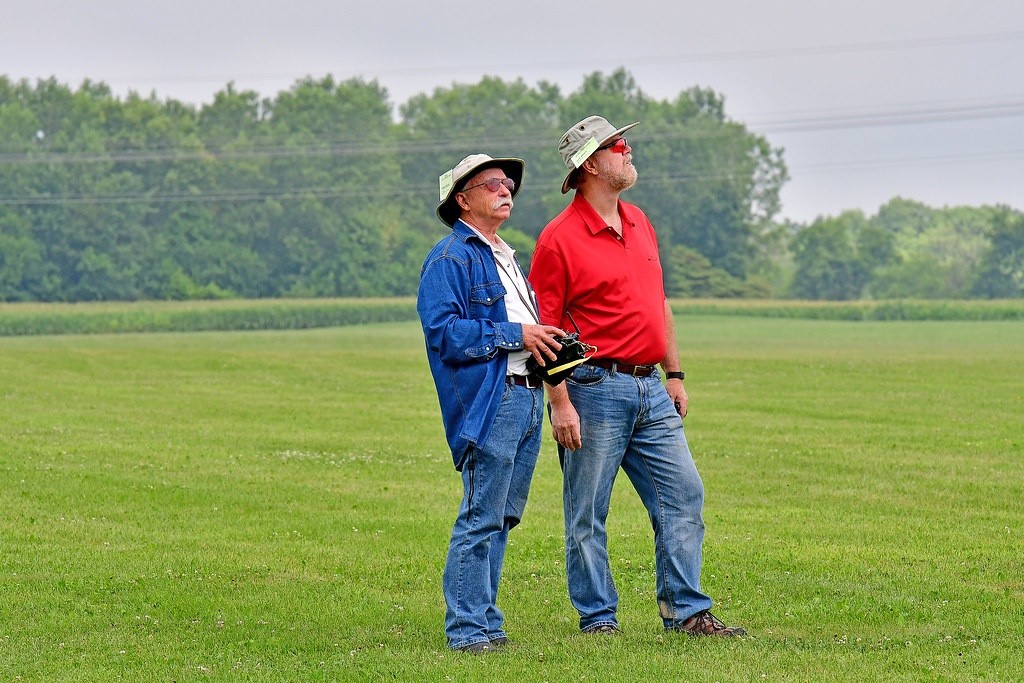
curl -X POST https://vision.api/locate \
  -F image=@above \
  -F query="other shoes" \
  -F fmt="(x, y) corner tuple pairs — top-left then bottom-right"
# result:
(494, 637), (510, 645)
(466, 643), (497, 652)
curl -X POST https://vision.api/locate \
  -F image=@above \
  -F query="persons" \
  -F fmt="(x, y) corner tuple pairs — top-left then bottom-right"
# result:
(526, 115), (746, 639)
(416, 153), (566, 653)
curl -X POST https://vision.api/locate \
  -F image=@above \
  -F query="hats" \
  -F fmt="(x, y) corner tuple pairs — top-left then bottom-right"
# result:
(557, 115), (639, 194)
(435, 153), (525, 229)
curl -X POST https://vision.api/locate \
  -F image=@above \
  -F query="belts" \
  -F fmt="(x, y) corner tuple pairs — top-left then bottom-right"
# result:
(506, 375), (542, 389)
(584, 359), (655, 377)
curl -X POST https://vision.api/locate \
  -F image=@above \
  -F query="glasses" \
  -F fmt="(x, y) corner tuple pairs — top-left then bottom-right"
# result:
(462, 178), (515, 192)
(596, 138), (627, 153)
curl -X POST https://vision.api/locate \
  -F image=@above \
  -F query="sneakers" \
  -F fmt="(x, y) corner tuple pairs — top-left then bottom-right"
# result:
(676, 609), (746, 636)
(588, 626), (615, 634)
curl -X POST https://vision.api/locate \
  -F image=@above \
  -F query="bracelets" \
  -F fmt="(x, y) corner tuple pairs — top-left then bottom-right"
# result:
(666, 372), (685, 380)
